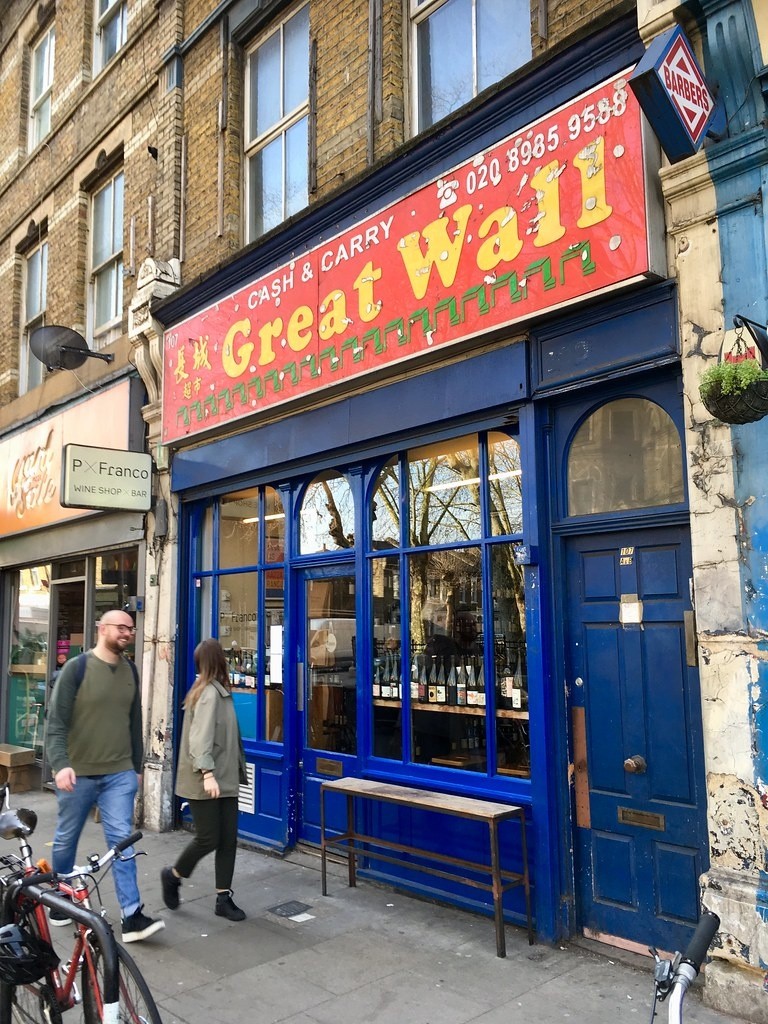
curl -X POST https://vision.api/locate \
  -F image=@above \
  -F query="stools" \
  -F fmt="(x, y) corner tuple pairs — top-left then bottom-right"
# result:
(433, 754), (485, 771)
(496, 763), (531, 776)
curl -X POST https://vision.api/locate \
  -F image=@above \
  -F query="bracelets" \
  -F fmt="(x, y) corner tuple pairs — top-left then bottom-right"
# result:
(201, 770), (212, 775)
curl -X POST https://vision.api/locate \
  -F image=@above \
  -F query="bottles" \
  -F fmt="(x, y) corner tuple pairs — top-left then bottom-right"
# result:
(419, 655), (429, 703)
(437, 656), (447, 704)
(229, 651), (236, 686)
(251, 651), (258, 689)
(446, 655), (457, 706)
(466, 655), (478, 707)
(241, 651), (252, 687)
(410, 654), (420, 702)
(373, 654), (402, 701)
(456, 619), (476, 634)
(265, 662), (270, 688)
(495, 658), (514, 710)
(428, 655), (439, 703)
(477, 657), (486, 708)
(456, 655), (469, 706)
(314, 666), (344, 686)
(512, 648), (528, 712)
(234, 652), (241, 687)
(459, 719), (486, 757)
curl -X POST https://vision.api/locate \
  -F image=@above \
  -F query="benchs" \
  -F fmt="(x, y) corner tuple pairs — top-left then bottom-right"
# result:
(0, 744), (37, 795)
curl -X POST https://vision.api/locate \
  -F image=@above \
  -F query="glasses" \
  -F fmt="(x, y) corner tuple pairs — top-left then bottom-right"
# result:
(103, 624), (138, 635)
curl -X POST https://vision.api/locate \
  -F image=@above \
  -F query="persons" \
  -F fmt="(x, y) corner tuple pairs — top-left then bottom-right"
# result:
(47, 610), (165, 942)
(161, 638), (248, 922)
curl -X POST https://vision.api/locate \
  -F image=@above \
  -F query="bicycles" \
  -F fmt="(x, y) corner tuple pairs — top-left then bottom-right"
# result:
(0, 783), (163, 1024)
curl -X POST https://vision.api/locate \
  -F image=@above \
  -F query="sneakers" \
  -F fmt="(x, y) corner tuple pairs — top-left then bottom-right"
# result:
(214, 891), (246, 921)
(48, 894), (72, 926)
(121, 903), (165, 943)
(161, 867), (181, 909)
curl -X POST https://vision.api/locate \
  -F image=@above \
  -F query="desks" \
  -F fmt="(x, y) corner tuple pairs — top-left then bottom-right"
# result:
(320, 777), (533, 959)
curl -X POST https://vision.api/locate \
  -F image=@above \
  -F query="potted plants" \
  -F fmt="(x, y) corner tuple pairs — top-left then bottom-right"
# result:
(698, 359), (768, 424)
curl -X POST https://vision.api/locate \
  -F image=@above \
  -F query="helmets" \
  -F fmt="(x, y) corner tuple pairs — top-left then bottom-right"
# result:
(0, 924), (60, 986)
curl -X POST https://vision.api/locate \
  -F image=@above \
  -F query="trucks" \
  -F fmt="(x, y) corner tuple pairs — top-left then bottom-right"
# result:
(308, 617), (359, 673)
